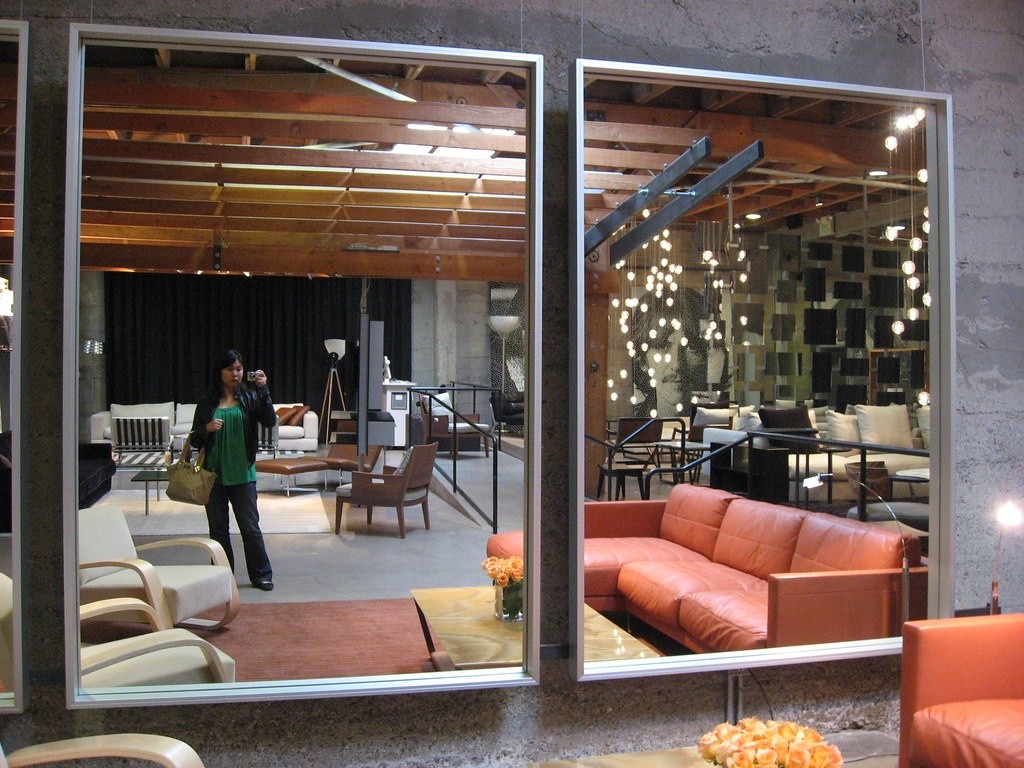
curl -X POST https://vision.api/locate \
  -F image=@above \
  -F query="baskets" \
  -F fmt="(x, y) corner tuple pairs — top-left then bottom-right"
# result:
(166, 430), (219, 505)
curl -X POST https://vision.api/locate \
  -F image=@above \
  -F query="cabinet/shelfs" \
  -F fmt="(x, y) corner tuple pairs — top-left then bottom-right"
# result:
(710, 440), (789, 505)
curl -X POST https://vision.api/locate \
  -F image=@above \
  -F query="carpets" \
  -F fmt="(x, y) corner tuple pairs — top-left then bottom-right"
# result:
(89, 489), (332, 536)
(120, 450), (305, 468)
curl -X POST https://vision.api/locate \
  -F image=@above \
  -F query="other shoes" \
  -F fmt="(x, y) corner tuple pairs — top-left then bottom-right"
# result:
(259, 580), (274, 591)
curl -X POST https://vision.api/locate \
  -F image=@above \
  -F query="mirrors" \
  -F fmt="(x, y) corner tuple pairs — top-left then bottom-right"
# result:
(0, 18), (29, 716)
(63, 22), (543, 708)
(572, 57), (953, 681)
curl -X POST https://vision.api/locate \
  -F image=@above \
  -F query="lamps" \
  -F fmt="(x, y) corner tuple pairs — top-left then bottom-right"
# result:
(486, 316), (522, 393)
(802, 473), (909, 625)
(816, 215), (834, 236)
(318, 339), (346, 447)
(885, 107), (932, 334)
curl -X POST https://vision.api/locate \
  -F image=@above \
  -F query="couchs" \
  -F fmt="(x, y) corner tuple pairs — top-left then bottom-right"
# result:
(0, 430), (116, 533)
(91, 402), (319, 451)
(612, 416), (930, 502)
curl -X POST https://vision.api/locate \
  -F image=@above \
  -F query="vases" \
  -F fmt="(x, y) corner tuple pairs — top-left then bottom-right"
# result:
(493, 579), (524, 622)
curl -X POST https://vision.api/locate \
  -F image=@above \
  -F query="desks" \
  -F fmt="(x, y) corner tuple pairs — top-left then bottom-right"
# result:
(897, 467), (930, 480)
(655, 441), (710, 483)
(367, 412), (395, 466)
(131, 471), (170, 515)
(82, 598), (449, 683)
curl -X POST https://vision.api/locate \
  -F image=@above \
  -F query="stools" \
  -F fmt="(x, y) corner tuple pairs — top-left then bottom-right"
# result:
(254, 458), (328, 498)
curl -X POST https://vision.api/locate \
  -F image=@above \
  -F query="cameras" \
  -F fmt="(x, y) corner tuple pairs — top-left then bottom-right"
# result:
(247, 372), (259, 381)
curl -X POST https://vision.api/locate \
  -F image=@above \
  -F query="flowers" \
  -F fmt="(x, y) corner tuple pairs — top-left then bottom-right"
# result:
(482, 557), (524, 619)
(699, 714), (843, 767)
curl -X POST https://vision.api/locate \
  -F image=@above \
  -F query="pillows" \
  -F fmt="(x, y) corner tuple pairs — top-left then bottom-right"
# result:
(276, 408), (297, 426)
(422, 392), (454, 420)
(693, 400), (931, 457)
(288, 406), (311, 425)
(394, 445), (413, 476)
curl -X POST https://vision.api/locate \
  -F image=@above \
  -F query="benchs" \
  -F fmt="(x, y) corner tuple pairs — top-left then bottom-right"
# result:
(487, 483), (930, 655)
(110, 417), (174, 464)
(257, 415), (280, 458)
(597, 463), (644, 501)
(877, 521), (928, 556)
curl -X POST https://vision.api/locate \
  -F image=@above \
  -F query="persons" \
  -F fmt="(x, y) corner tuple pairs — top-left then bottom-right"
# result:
(189, 349), (277, 591)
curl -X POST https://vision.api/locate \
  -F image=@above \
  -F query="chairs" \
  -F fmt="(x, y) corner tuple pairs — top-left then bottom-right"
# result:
(78, 503), (241, 630)
(610, 417), (663, 468)
(77, 596), (235, 687)
(0, 734), (205, 767)
(672, 402), (733, 443)
(898, 612), (1024, 768)
(419, 392), (489, 461)
(294, 445), (386, 487)
(335, 442), (439, 539)
(0, 570), (15, 694)
(847, 461), (929, 522)
(488, 392), (524, 432)
